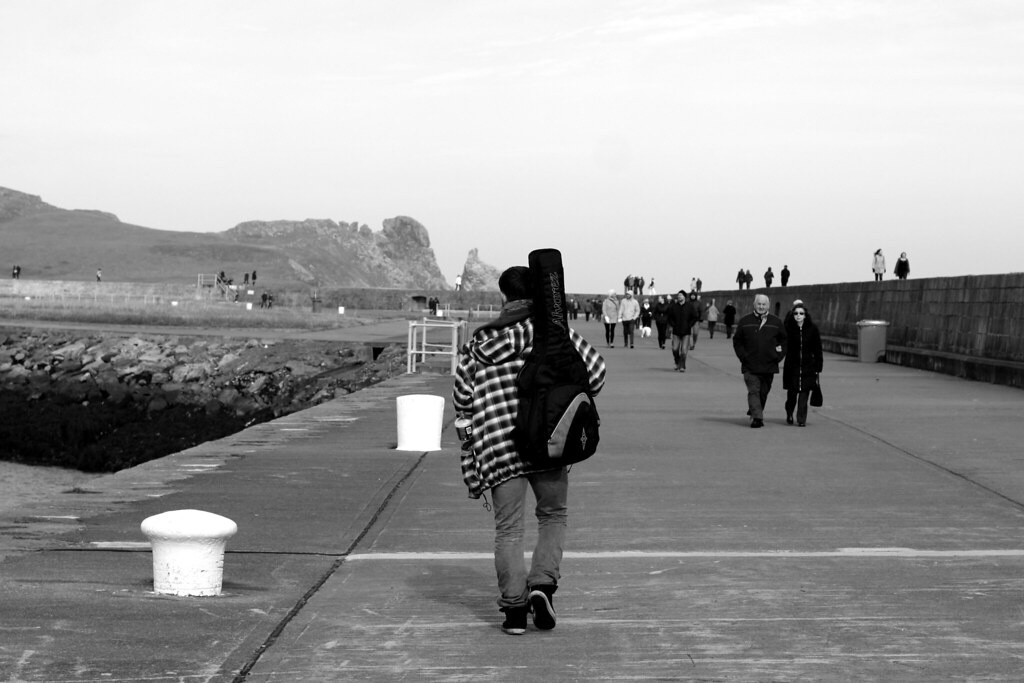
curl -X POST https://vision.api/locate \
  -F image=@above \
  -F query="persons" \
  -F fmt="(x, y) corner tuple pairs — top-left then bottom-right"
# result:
(764, 265), (790, 288)
(736, 268), (753, 291)
(696, 278), (702, 292)
(668, 290), (696, 372)
(428, 274), (602, 321)
(648, 294), (678, 304)
(690, 278), (696, 292)
(218, 267), (275, 310)
(732, 294), (823, 428)
(602, 290), (619, 349)
(641, 296), (670, 349)
(95, 268), (103, 282)
(12, 264), (22, 280)
(452, 266), (606, 636)
(618, 290), (640, 348)
(688, 289), (703, 350)
(894, 252), (910, 279)
(706, 299), (736, 339)
(624, 275), (656, 295)
(872, 249), (886, 281)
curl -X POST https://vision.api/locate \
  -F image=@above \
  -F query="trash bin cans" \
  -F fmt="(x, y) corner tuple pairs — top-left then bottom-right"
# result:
(311, 297), (322, 313)
(856, 320), (890, 364)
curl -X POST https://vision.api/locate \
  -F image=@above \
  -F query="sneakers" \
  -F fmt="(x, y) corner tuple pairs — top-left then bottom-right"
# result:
(496, 605), (530, 636)
(529, 583), (558, 631)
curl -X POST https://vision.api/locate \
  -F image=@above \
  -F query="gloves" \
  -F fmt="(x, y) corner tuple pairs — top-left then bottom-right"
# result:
(618, 319), (622, 323)
(872, 268), (875, 273)
(605, 316), (609, 323)
(884, 269), (886, 273)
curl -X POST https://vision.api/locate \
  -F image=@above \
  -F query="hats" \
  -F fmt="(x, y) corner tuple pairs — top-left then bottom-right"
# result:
(608, 289), (616, 296)
(643, 298), (649, 303)
(793, 300), (802, 305)
(678, 290), (687, 297)
(626, 290), (633, 296)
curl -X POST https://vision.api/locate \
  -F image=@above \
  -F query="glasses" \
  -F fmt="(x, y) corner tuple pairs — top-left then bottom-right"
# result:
(793, 312), (805, 315)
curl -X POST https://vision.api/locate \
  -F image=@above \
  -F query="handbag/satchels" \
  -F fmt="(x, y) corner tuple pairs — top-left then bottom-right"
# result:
(810, 372), (823, 407)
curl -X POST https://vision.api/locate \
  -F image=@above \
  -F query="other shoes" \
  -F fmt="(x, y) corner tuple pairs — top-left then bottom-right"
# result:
(747, 410), (752, 416)
(673, 364), (679, 371)
(798, 423), (806, 426)
(787, 415), (794, 424)
(750, 418), (764, 428)
(680, 367), (685, 372)
(610, 343), (614, 348)
(606, 343), (609, 348)
(662, 344), (666, 349)
(624, 343), (628, 348)
(630, 344), (634, 348)
